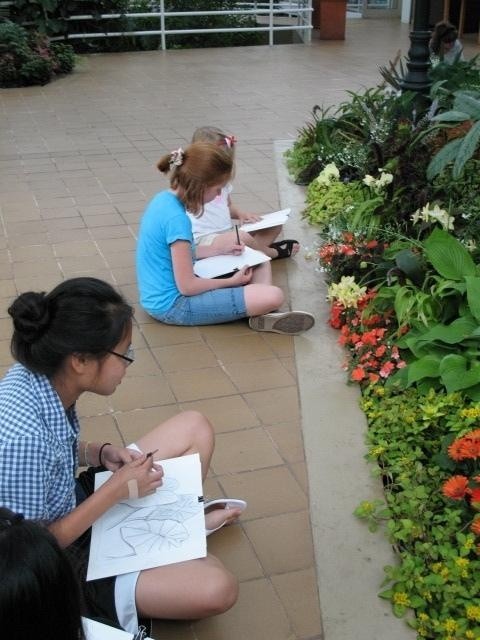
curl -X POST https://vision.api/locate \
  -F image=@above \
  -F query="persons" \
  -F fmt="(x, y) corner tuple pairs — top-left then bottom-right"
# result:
(135, 142), (316, 334)
(186, 125), (300, 260)
(429, 21), (465, 69)
(1, 507), (85, 639)
(0, 277), (249, 624)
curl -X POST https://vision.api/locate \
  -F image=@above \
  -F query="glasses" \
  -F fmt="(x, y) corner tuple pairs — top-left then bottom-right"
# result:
(107, 349), (135, 365)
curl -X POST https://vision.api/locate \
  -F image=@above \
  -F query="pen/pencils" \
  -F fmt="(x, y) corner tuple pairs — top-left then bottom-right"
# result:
(234, 225), (241, 252)
(146, 448), (159, 459)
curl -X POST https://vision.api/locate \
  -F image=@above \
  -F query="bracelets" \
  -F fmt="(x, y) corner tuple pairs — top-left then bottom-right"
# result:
(83, 440), (93, 468)
(99, 442), (110, 469)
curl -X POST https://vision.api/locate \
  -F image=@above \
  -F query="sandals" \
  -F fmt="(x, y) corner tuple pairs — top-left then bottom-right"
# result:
(205, 498), (246, 538)
(270, 239), (299, 261)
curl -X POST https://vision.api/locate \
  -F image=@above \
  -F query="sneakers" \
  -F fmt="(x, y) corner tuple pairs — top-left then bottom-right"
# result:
(249, 312), (315, 334)
